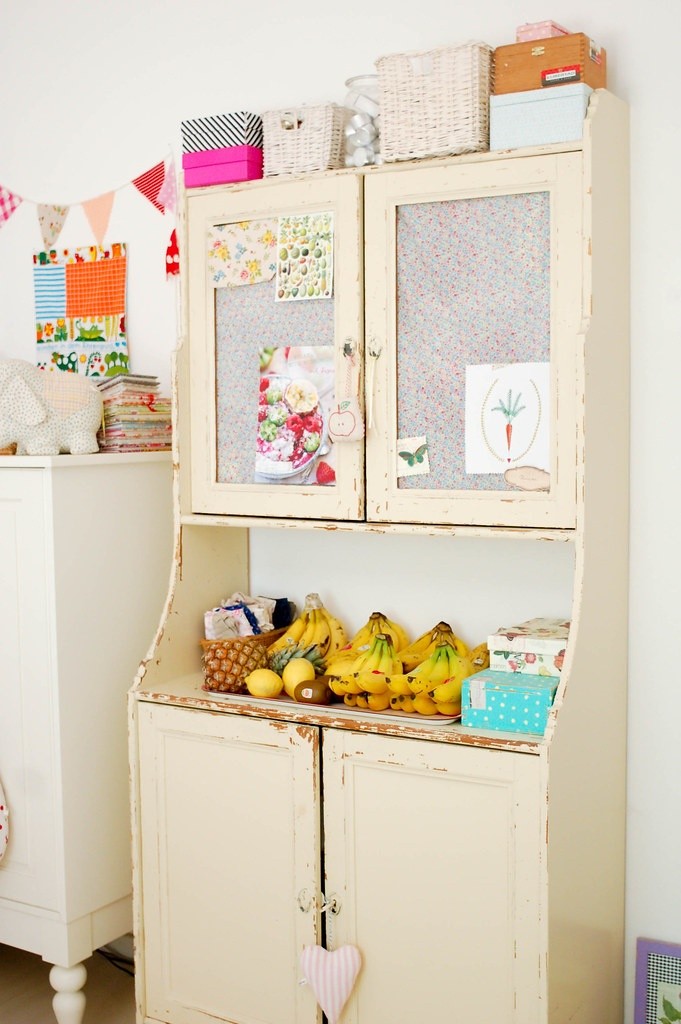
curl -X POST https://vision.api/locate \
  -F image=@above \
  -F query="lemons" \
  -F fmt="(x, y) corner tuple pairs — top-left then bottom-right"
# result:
(282, 658), (315, 700)
(244, 669), (284, 698)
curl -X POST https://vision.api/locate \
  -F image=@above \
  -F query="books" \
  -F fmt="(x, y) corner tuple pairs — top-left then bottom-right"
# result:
(96, 375), (173, 452)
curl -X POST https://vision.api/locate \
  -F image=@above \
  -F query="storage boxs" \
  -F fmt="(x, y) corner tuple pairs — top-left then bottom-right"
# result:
(516, 20), (572, 43)
(489, 82), (593, 150)
(461, 668), (560, 736)
(180, 111), (264, 154)
(487, 616), (572, 677)
(182, 147), (263, 187)
(494, 32), (607, 95)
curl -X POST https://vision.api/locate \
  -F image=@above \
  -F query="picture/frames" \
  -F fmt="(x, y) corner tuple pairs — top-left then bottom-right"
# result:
(633, 937), (681, 1024)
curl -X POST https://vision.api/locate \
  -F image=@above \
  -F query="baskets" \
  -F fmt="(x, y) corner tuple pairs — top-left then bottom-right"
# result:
(200, 627), (299, 669)
(375, 42), (494, 162)
(261, 104), (356, 176)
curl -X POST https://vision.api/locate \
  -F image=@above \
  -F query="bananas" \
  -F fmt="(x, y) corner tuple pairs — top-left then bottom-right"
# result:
(323, 612), (489, 716)
(265, 593), (346, 669)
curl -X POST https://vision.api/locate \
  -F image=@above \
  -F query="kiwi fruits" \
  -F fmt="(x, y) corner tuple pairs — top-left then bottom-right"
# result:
(294, 675), (342, 705)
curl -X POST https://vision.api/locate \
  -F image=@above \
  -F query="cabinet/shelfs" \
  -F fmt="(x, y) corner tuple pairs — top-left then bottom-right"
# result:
(128, 88), (629, 1024)
(0, 451), (173, 1024)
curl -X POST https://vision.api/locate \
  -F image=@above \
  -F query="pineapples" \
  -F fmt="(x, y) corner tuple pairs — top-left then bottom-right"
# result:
(200, 638), (327, 692)
